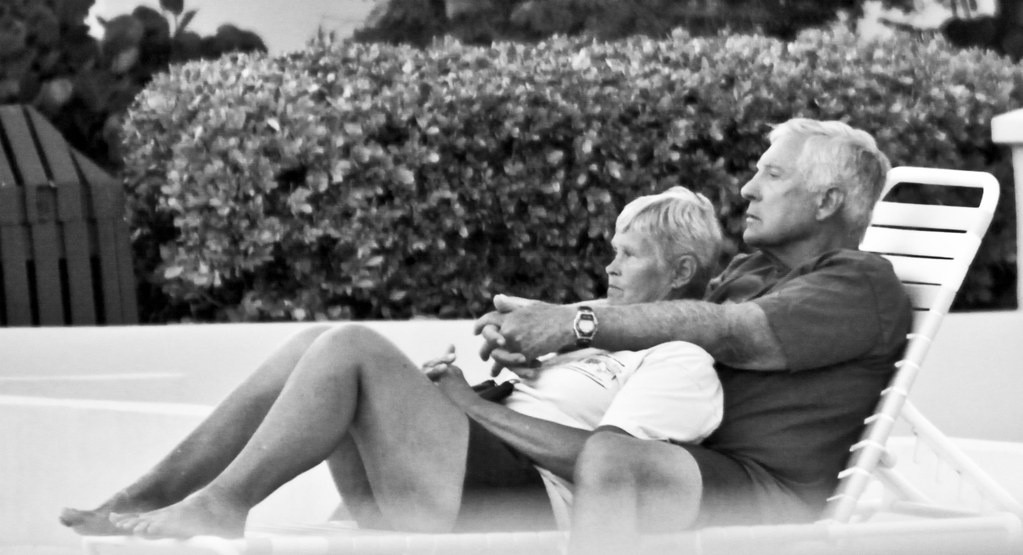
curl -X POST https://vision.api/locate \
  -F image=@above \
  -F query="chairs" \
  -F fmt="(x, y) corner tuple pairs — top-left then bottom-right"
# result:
(76, 164), (1023, 555)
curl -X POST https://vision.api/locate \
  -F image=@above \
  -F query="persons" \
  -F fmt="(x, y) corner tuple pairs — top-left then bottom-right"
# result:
(57, 181), (725, 540)
(470, 114), (910, 540)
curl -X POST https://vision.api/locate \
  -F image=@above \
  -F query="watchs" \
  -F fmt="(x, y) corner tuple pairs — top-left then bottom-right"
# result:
(571, 306), (598, 350)
